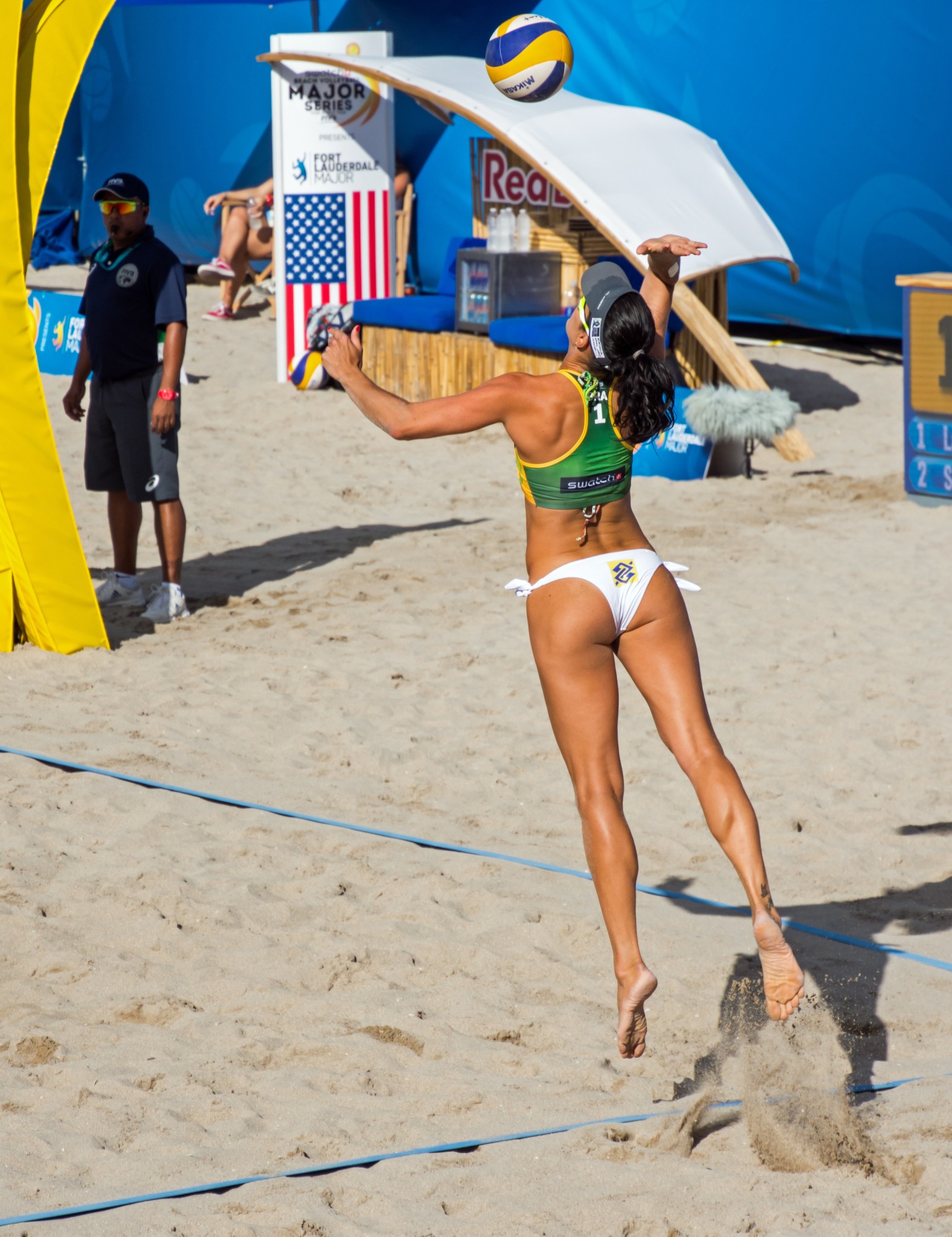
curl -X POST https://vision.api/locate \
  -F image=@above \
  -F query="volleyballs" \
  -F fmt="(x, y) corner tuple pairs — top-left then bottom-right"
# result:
(288, 350), (330, 391)
(486, 12), (574, 103)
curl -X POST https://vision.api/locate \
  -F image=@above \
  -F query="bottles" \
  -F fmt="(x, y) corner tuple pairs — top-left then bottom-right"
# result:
(516, 208), (530, 252)
(245, 196), (262, 230)
(563, 279), (578, 317)
(486, 206), (517, 254)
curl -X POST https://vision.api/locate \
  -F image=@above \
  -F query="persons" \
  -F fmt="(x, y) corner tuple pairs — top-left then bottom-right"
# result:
(197, 177), (274, 320)
(62, 174), (188, 624)
(320, 235), (805, 1059)
(395, 164), (411, 209)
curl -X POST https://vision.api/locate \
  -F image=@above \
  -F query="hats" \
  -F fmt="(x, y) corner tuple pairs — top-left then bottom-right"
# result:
(93, 173), (150, 206)
(580, 262), (642, 371)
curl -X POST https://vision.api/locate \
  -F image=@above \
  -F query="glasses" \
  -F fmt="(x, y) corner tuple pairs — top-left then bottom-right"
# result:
(578, 296), (600, 338)
(98, 200), (145, 215)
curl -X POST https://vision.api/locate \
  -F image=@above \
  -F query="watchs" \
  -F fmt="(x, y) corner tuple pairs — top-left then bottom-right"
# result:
(158, 388), (179, 400)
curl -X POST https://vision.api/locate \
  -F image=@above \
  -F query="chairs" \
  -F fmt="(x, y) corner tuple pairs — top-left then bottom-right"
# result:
(353, 238), (487, 402)
(219, 183), (413, 320)
(489, 256), (691, 379)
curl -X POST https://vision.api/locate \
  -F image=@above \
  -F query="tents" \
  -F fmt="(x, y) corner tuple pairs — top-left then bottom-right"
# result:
(17, 0), (952, 340)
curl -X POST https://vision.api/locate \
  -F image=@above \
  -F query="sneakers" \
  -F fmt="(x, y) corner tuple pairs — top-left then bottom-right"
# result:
(97, 570), (146, 607)
(197, 259), (235, 283)
(201, 301), (233, 321)
(140, 582), (191, 624)
